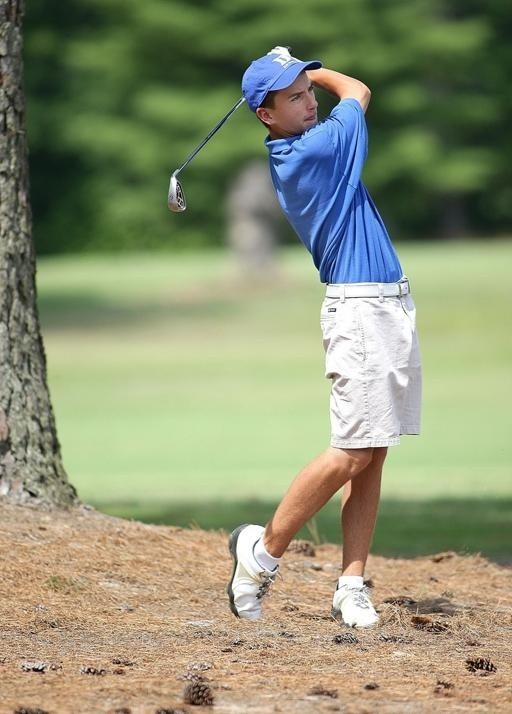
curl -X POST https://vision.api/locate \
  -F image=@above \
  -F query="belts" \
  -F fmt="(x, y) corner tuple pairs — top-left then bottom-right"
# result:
(324, 276), (412, 300)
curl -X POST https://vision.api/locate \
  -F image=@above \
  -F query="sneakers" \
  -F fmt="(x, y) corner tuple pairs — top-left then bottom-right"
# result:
(330, 581), (381, 629)
(226, 522), (280, 620)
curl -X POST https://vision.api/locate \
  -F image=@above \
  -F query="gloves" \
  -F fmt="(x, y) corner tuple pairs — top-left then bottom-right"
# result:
(267, 45), (291, 58)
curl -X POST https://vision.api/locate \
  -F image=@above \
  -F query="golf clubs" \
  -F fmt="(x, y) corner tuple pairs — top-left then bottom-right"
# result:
(167, 46), (293, 213)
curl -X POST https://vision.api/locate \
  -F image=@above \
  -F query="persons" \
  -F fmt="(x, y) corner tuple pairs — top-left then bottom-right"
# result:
(221, 42), (427, 629)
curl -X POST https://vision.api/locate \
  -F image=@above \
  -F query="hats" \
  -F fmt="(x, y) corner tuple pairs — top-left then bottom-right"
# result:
(241, 52), (323, 114)
